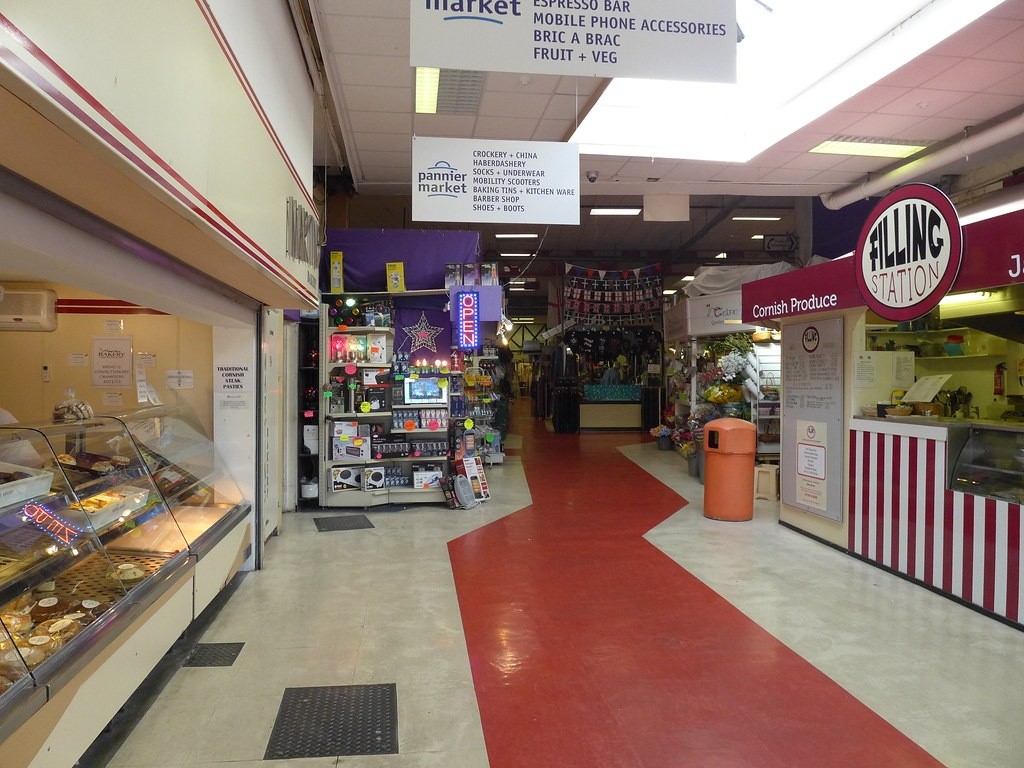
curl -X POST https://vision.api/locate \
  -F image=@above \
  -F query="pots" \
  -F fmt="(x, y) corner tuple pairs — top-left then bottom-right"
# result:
(300, 479), (318, 498)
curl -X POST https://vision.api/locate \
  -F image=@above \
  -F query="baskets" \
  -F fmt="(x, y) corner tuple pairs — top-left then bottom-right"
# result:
(885, 408), (913, 416)
(916, 402), (944, 416)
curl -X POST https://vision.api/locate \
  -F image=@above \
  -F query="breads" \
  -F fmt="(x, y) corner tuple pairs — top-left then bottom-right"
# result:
(56, 454), (130, 472)
(53, 401), (93, 423)
(0, 564), (144, 697)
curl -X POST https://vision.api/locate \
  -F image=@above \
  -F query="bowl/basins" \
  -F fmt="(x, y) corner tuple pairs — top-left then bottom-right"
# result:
(945, 344), (965, 356)
(920, 344), (943, 357)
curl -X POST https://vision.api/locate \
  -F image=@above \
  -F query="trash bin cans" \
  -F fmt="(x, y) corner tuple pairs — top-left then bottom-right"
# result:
(702, 418), (757, 521)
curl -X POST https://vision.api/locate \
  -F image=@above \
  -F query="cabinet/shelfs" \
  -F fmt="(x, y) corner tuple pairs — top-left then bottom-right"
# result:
(296, 318), (320, 504)
(458, 349), (505, 466)
(318, 291), (452, 510)
(661, 333), (781, 464)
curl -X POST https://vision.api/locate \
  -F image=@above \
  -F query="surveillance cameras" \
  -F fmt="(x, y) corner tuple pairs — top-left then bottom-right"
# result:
(586, 170), (598, 183)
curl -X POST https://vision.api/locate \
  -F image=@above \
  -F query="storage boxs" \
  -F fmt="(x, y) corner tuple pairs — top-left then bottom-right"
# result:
(332, 419), (491, 511)
(330, 334), (388, 364)
(304, 425), (318, 454)
(386, 263), (406, 291)
(331, 251), (343, 294)
(483, 432), (500, 454)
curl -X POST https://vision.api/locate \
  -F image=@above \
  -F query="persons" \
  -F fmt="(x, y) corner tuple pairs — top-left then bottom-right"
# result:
(0, 408), (45, 486)
(601, 361), (621, 386)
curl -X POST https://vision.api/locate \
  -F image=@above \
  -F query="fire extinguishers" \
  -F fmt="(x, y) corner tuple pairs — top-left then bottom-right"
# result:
(993, 361), (1007, 395)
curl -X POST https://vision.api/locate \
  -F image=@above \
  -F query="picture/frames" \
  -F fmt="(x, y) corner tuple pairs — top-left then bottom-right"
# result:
(404, 378), (449, 405)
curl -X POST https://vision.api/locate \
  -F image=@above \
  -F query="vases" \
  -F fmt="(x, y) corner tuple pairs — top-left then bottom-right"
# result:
(657, 439), (672, 450)
(688, 453), (698, 476)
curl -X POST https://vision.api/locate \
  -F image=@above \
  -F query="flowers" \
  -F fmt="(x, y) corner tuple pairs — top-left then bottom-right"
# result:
(650, 334), (751, 452)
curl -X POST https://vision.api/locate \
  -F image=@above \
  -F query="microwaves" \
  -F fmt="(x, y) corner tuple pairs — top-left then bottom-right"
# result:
(344, 445), (366, 460)
